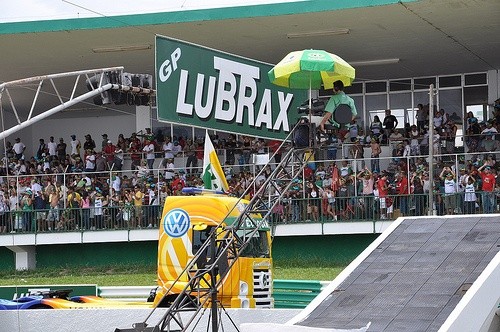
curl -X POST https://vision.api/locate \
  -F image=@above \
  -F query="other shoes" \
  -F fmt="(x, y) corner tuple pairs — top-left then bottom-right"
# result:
(154, 224), (157, 227)
(332, 215), (337, 221)
(90, 226), (95, 230)
(306, 220), (311, 222)
(10, 231), (16, 233)
(147, 224), (152, 228)
(380, 214), (386, 219)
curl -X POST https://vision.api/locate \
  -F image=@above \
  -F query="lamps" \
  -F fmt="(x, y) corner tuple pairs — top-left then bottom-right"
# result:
(85, 72), (152, 106)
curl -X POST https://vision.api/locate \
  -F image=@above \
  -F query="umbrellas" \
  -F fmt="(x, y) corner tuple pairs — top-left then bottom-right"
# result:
(267, 48), (355, 147)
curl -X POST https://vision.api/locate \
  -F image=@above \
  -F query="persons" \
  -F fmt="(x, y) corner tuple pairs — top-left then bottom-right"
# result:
(319, 79), (358, 131)
(210, 99), (500, 224)
(0, 128), (205, 235)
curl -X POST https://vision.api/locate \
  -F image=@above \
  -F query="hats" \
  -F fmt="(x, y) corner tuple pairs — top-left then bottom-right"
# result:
(102, 134), (107, 136)
(107, 141), (111, 143)
(145, 128), (150, 130)
(71, 135), (75, 137)
(15, 138), (20, 140)
(85, 134), (91, 137)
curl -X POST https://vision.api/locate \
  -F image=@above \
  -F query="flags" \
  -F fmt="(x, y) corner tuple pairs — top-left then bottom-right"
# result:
(200, 128), (229, 193)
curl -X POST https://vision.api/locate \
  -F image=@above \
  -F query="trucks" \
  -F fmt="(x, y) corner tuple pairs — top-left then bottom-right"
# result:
(1, 187), (275, 309)
